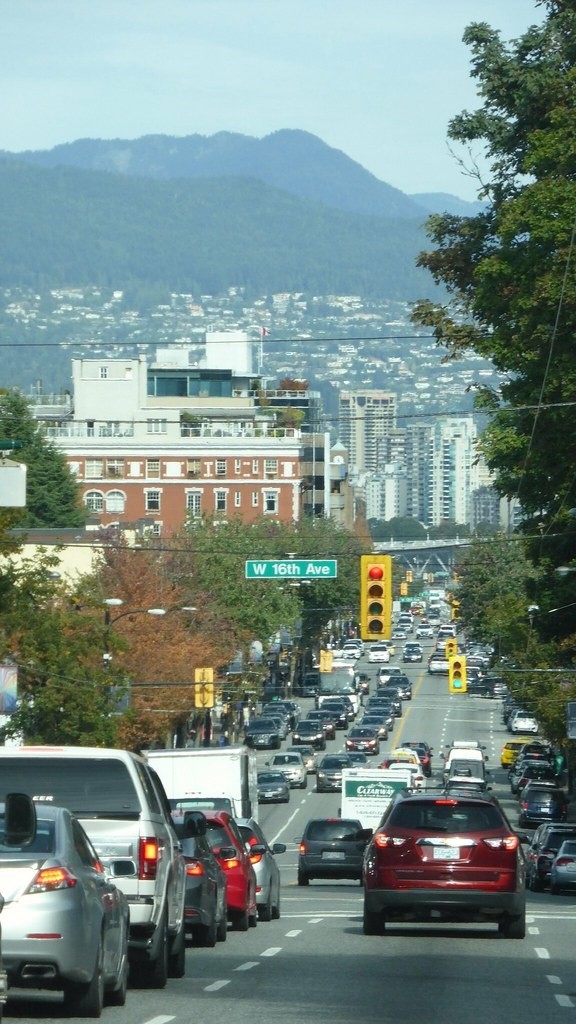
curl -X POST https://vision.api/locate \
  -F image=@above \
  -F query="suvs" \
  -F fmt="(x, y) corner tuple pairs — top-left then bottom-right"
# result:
(529, 827), (576, 892)
(522, 823), (576, 889)
(354, 787), (525, 939)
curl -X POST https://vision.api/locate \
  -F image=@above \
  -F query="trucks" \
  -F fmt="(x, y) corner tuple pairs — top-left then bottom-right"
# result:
(140, 747), (259, 825)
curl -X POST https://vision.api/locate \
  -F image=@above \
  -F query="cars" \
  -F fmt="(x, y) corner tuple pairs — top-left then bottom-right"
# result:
(342, 604), (458, 675)
(171, 816), (227, 948)
(243, 666), (433, 803)
(1, 802), (130, 1018)
(438, 740), (493, 796)
(205, 818), (287, 921)
(172, 810), (267, 931)
(547, 840), (576, 895)
(293, 819), (374, 887)
(501, 737), (571, 828)
(465, 642), (539, 736)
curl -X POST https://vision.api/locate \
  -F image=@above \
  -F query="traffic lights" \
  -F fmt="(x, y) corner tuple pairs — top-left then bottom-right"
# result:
(449, 655), (467, 693)
(446, 638), (457, 660)
(361, 555), (392, 640)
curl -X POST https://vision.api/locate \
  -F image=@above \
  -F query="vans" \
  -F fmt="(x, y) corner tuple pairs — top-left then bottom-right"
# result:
(0, 746), (187, 988)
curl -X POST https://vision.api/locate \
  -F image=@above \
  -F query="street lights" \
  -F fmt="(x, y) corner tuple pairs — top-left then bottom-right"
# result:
(102, 597), (197, 749)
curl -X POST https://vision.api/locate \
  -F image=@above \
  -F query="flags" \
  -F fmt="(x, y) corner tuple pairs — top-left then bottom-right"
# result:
(262, 327), (271, 336)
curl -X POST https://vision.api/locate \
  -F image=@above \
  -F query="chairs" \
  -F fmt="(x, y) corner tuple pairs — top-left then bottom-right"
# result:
(467, 813), (488, 833)
(398, 808), (421, 829)
